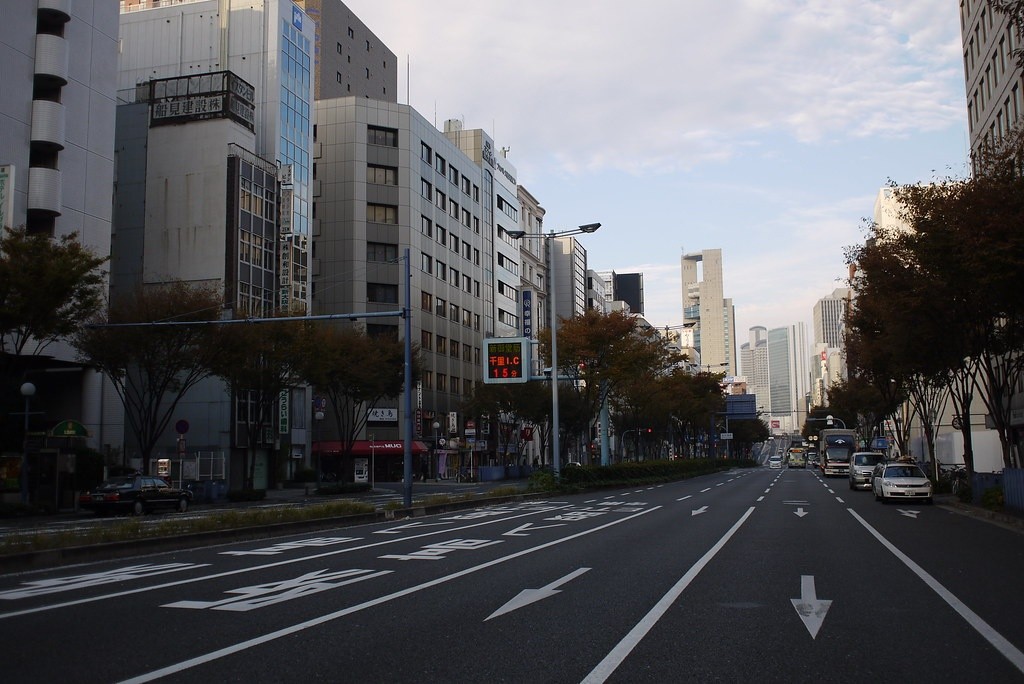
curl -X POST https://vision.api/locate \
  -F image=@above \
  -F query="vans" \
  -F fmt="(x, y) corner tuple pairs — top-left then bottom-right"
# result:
(768, 456), (784, 469)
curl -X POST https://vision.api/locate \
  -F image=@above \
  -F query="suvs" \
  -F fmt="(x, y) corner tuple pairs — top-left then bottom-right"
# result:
(849, 452), (888, 490)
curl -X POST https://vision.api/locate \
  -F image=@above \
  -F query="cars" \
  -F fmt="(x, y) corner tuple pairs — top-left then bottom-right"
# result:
(869, 459), (934, 504)
(812, 456), (819, 468)
(78, 474), (194, 518)
(564, 462), (581, 468)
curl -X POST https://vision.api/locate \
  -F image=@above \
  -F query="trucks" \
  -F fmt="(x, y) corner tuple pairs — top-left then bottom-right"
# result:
(819, 428), (859, 477)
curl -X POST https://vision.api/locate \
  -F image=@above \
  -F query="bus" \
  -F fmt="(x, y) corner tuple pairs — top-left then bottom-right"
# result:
(786, 447), (809, 469)
(807, 451), (817, 465)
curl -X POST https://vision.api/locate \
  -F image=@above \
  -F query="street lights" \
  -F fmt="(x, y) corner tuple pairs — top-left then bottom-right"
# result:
(688, 362), (730, 374)
(637, 321), (696, 458)
(432, 422), (440, 482)
(313, 411), (325, 490)
(19, 383), (38, 461)
(504, 223), (605, 484)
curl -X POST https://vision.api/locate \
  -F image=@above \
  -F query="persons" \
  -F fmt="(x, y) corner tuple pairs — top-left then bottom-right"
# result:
(532, 455), (540, 469)
